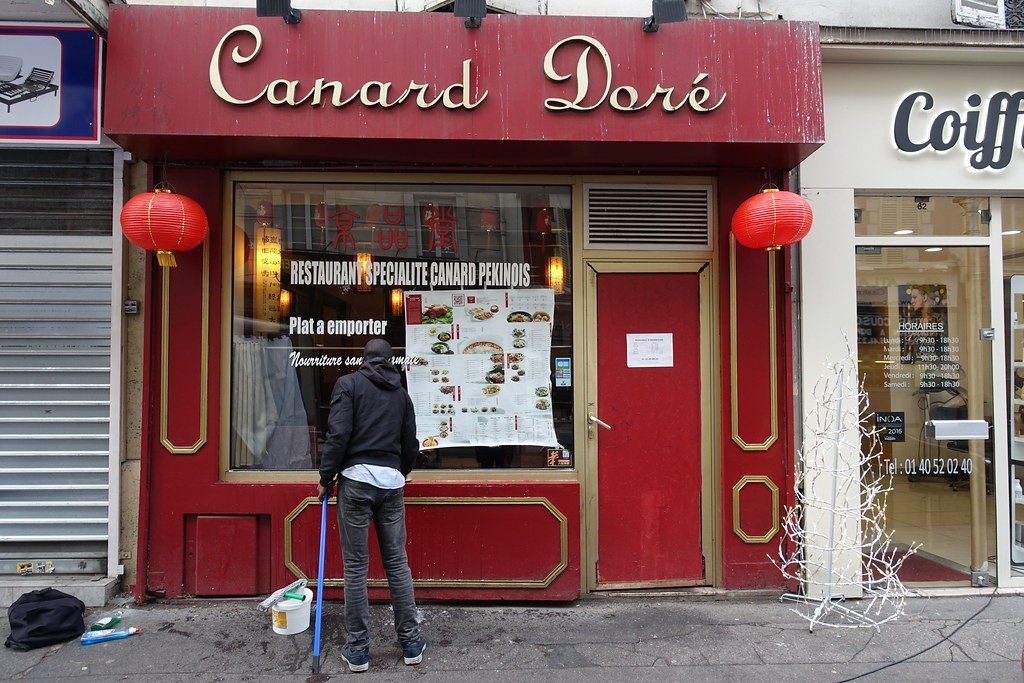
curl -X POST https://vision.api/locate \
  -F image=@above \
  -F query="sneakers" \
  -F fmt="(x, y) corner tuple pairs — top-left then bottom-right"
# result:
(402, 639), (427, 666)
(341, 644), (369, 672)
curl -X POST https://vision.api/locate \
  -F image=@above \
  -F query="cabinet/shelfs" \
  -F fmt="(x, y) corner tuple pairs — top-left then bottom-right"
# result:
(1003, 273), (1024, 565)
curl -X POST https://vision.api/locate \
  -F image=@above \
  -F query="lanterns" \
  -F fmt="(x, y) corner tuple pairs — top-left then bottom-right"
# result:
(316, 204), (330, 246)
(256, 202), (272, 226)
(732, 185), (815, 253)
(278, 289), (292, 317)
(480, 206), (498, 251)
(424, 206), (434, 225)
(390, 288), (403, 316)
(537, 208), (551, 253)
(119, 184), (207, 266)
(544, 255), (568, 296)
(366, 204), (382, 252)
(353, 251), (374, 292)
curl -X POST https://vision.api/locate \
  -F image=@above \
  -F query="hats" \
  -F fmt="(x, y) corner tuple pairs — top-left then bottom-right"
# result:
(364, 339), (394, 359)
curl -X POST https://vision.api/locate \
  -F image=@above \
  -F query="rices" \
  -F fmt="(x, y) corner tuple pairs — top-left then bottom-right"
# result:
(465, 345), (502, 354)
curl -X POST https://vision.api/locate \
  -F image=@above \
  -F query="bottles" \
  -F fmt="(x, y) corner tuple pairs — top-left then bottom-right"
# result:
(1014, 479), (1023, 504)
(91, 610), (124, 631)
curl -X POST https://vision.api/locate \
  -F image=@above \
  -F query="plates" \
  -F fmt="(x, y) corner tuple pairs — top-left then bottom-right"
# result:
(440, 420), (448, 438)
(482, 352), (526, 396)
(535, 386), (550, 410)
(430, 332), (451, 354)
(458, 335), (504, 355)
(422, 439), (438, 447)
(511, 329), (526, 348)
(506, 311), (551, 322)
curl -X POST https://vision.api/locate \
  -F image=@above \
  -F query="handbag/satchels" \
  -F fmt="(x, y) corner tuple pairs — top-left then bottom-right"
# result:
(5, 587), (85, 652)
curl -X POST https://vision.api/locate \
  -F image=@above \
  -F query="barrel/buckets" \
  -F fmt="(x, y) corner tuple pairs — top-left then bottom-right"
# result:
(272, 587), (313, 635)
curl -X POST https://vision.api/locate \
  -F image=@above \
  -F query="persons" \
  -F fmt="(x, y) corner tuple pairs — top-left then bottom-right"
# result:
(317, 339), (426, 672)
(910, 284), (942, 338)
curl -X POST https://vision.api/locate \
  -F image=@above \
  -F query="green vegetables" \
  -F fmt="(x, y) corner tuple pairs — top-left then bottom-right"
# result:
(422, 305), (453, 324)
(433, 331), (450, 350)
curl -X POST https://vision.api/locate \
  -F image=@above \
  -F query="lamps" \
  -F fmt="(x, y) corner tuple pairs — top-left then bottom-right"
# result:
(643, 0), (688, 33)
(544, 256), (566, 295)
(255, 227), (282, 333)
(256, 0), (301, 24)
(454, 0), (487, 28)
